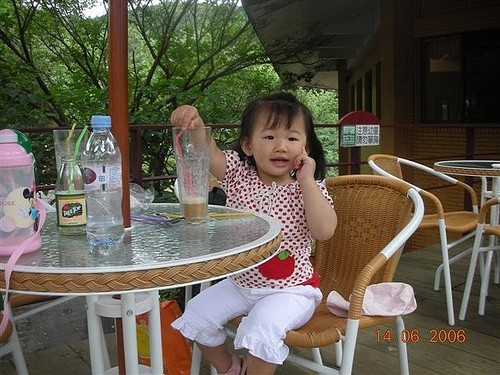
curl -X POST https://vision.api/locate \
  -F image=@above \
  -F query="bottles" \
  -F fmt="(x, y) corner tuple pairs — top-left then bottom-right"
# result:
(55, 157), (87, 240)
(83, 115), (125, 247)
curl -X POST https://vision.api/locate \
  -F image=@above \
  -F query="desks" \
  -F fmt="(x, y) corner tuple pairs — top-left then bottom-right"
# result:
(0, 203), (283, 375)
(433, 161), (500, 291)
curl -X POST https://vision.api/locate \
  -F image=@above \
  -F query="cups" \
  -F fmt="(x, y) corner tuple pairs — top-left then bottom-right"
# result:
(0, 129), (42, 255)
(53, 129), (88, 177)
(172, 126), (212, 221)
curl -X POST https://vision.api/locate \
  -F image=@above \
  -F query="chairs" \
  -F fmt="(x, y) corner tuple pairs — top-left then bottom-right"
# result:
(0, 198), (56, 375)
(368, 154), (500, 327)
(175, 174), (426, 375)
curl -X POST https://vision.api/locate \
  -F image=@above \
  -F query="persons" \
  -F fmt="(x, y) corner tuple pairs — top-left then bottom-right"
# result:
(170, 93), (339, 375)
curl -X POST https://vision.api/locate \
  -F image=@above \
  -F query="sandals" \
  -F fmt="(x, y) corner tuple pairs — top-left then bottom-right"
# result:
(217, 355), (248, 375)
